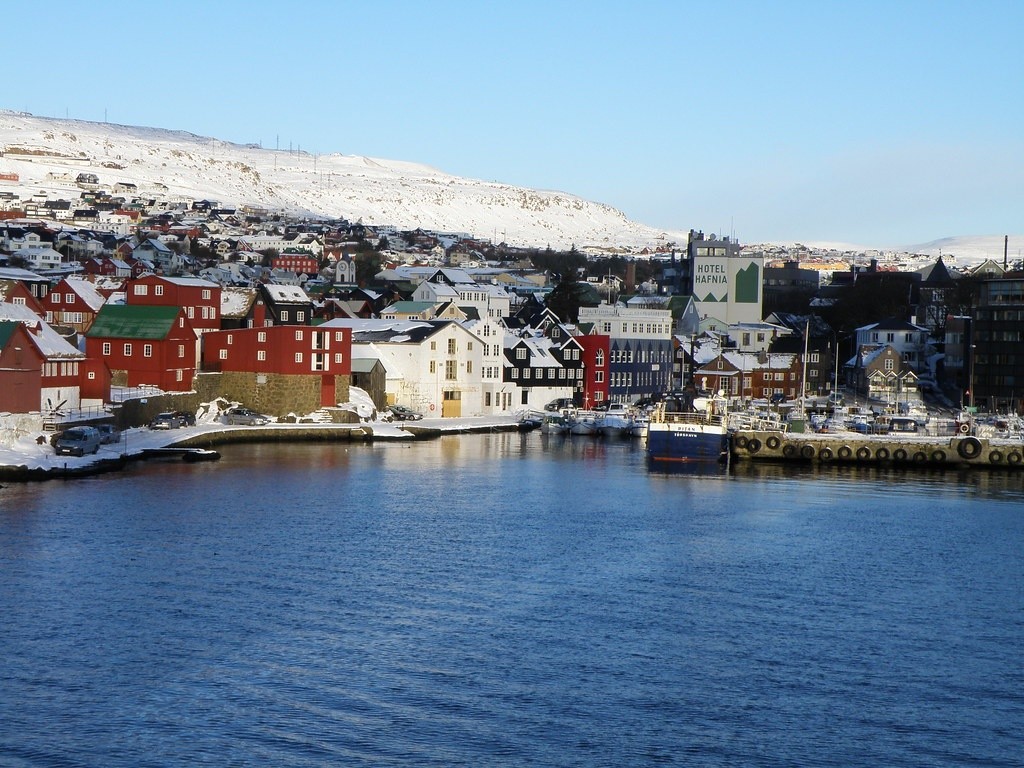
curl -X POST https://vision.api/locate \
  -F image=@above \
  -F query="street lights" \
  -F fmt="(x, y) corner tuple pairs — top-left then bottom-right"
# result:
(902, 354), (910, 416)
(767, 354), (771, 421)
(969, 344), (977, 436)
(681, 341), (685, 391)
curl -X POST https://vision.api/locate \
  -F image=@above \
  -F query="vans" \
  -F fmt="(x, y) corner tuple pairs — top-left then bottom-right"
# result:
(55, 425), (101, 457)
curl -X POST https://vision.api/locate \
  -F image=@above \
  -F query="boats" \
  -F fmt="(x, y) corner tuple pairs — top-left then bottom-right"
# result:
(514, 320), (1023, 471)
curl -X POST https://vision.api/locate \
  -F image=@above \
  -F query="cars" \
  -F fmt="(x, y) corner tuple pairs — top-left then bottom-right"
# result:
(382, 405), (423, 421)
(227, 406), (270, 426)
(174, 410), (195, 427)
(148, 412), (182, 431)
(94, 424), (122, 445)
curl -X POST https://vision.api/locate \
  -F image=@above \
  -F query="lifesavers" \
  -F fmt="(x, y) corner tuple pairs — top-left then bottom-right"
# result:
(876, 447), (891, 461)
(838, 445), (853, 460)
(747, 438), (762, 453)
(819, 447), (834, 462)
(894, 448), (909, 463)
(766, 436), (781, 449)
(1007, 452), (1022, 466)
(801, 445), (816, 460)
(856, 446), (872, 462)
(931, 449), (947, 464)
(913, 451), (928, 466)
(989, 451), (1004, 465)
(783, 444), (797, 459)
(957, 436), (983, 458)
(960, 424), (969, 433)
(736, 436), (749, 449)
